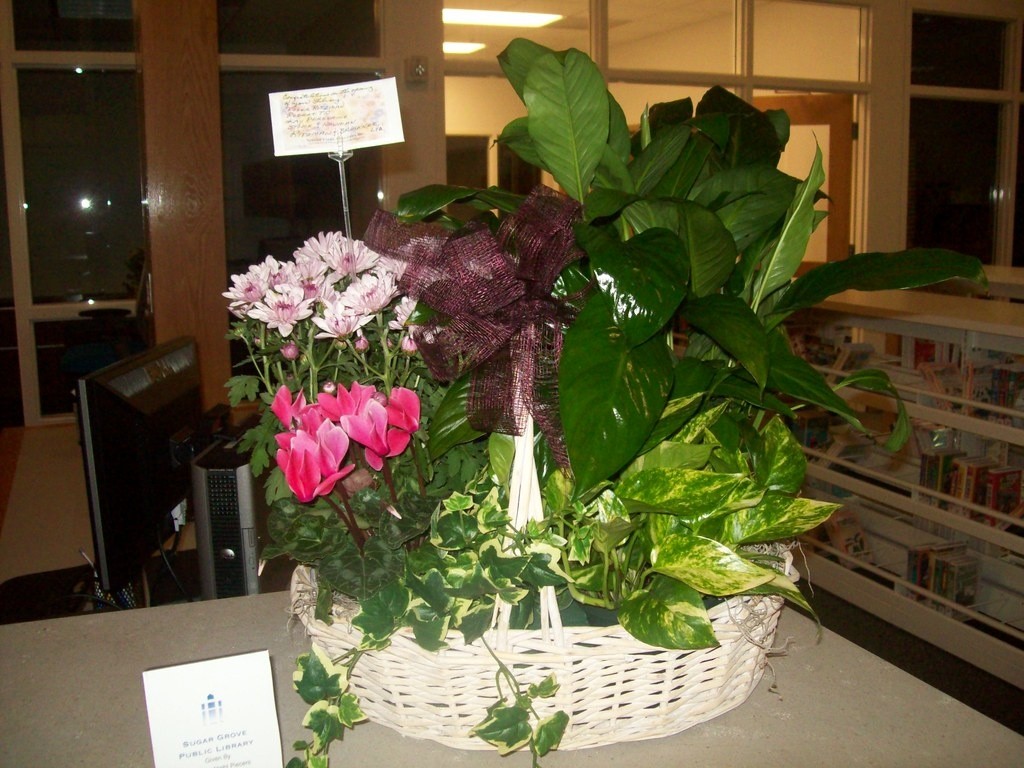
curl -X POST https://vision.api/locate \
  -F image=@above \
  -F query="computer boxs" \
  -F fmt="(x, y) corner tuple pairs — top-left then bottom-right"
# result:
(192, 409), (277, 599)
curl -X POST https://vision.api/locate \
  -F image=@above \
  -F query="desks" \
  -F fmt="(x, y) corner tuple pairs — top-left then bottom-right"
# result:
(0, 584), (1024, 768)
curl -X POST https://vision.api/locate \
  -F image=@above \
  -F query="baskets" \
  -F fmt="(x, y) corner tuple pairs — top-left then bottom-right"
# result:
(289, 304), (798, 753)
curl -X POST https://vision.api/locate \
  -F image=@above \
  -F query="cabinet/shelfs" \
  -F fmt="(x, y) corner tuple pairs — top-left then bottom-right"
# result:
(746, 265), (1024, 687)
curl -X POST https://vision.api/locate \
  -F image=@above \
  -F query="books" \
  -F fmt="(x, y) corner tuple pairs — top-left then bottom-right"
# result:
(782, 409), (859, 470)
(861, 409), (1010, 467)
(906, 542), (981, 621)
(805, 509), (874, 568)
(916, 358), (1024, 431)
(788, 332), (874, 386)
(917, 447), (1024, 535)
(911, 514), (998, 556)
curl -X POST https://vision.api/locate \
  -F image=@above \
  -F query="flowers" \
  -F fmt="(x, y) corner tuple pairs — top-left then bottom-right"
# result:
(221, 226), (438, 565)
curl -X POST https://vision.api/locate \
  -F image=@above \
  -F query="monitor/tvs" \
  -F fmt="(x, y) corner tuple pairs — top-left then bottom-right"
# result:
(76, 335), (211, 607)
